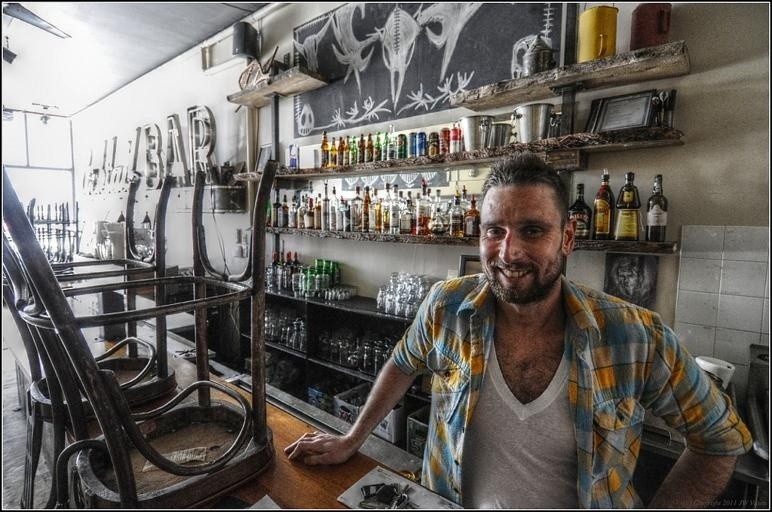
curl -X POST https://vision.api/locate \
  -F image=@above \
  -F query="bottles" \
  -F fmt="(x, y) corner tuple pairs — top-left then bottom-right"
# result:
(614, 171), (642, 240)
(320, 123), (398, 165)
(646, 172), (668, 241)
(567, 182), (593, 242)
(594, 173), (614, 238)
(271, 251), (341, 293)
(288, 144), (298, 170)
(272, 182), (483, 236)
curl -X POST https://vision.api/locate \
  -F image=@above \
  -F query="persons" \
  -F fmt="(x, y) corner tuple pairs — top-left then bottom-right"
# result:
(281, 152), (756, 510)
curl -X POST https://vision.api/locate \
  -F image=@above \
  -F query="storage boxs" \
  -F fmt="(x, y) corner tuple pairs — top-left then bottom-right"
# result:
(301, 364), (428, 457)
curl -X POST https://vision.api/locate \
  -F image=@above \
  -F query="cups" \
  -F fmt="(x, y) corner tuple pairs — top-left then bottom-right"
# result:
(376, 271), (433, 319)
(577, 5), (618, 64)
(318, 324), (401, 366)
(324, 284), (358, 300)
(264, 307), (307, 353)
(292, 273), (305, 296)
(628, 2), (672, 50)
(692, 355), (736, 393)
(266, 267), (282, 292)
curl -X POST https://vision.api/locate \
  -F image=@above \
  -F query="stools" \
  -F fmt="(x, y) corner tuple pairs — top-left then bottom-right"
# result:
(2, 201), (83, 277)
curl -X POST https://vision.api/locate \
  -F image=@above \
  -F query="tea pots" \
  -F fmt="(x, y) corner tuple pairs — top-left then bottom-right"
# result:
(521, 29), (557, 76)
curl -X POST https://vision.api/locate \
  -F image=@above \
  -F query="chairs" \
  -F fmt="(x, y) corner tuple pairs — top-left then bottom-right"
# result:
(2, 160), (278, 510)
(1, 172), (176, 511)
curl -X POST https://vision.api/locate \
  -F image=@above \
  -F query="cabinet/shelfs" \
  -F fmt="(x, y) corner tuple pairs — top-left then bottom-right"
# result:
(223, 265), (308, 404)
(306, 289), (430, 456)
(228, 40), (691, 396)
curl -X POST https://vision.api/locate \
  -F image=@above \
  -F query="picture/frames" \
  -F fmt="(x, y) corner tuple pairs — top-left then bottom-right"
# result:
(459, 254), (487, 280)
(585, 89), (657, 134)
